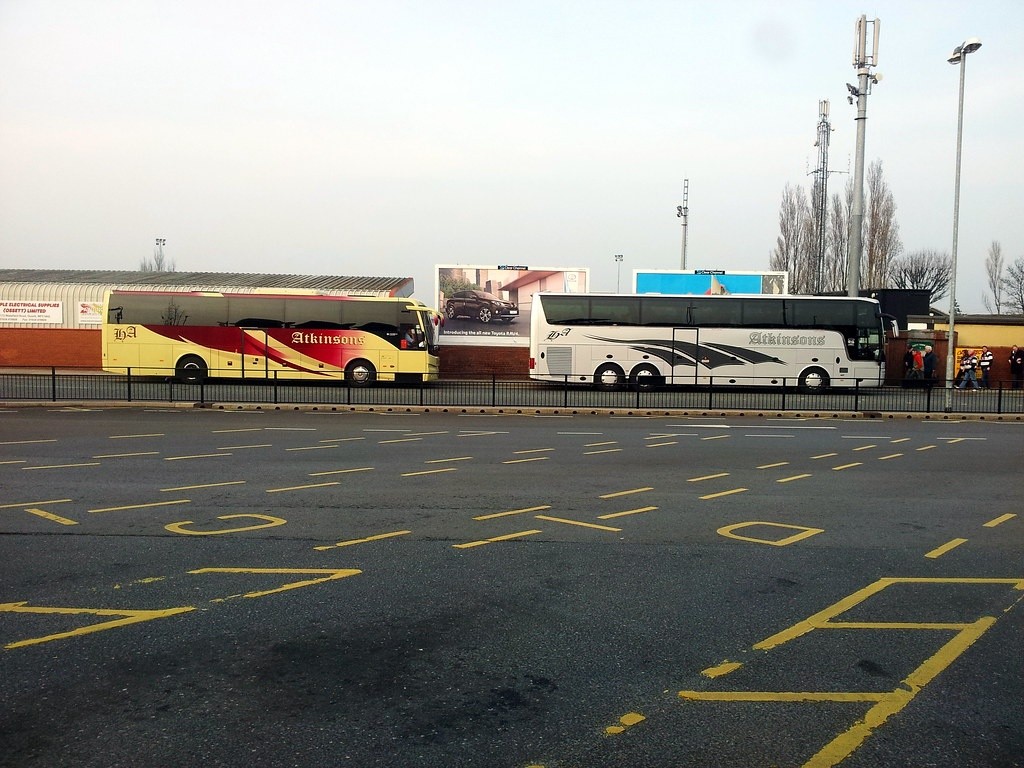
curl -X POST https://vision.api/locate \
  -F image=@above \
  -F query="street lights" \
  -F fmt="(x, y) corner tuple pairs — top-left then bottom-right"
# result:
(944, 38), (982, 413)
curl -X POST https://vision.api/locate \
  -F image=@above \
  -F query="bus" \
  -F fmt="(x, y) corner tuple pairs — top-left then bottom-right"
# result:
(102, 290), (445, 388)
(528, 292), (900, 394)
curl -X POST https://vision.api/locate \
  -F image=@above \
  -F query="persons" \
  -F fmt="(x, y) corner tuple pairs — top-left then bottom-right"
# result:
(903, 343), (1024, 392)
(405, 328), (415, 347)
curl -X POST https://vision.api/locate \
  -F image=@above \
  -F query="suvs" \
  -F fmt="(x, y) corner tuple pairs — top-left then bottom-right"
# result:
(446, 290), (520, 324)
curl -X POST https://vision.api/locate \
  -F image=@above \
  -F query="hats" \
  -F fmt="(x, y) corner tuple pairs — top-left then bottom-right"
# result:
(907, 344), (912, 349)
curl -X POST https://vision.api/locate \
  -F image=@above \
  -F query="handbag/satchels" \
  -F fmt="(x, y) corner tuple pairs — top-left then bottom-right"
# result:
(924, 374), (940, 388)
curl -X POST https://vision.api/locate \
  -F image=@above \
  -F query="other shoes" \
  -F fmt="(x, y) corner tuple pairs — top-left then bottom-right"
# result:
(972, 390), (978, 392)
(956, 387), (959, 388)
